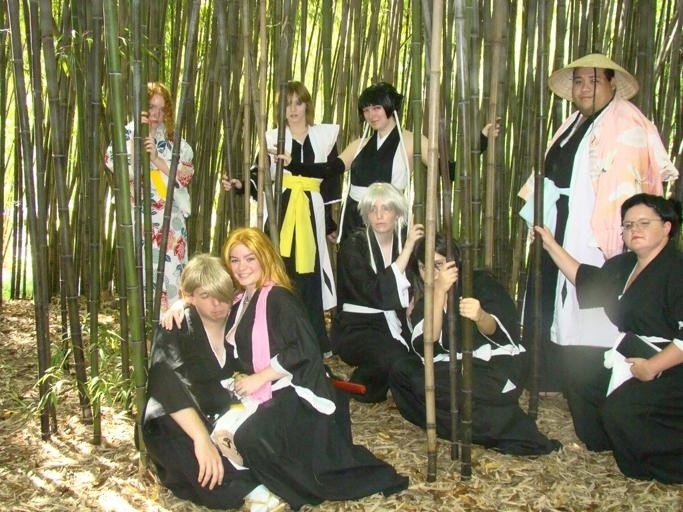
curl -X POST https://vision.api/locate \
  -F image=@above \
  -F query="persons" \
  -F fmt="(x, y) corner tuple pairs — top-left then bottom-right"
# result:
(221, 78), (339, 358)
(103, 84), (196, 316)
(530, 195), (683, 484)
(328, 184), (426, 406)
(211, 226), (409, 510)
(387, 234), (563, 454)
(276, 82), (504, 355)
(144, 255), (291, 512)
(518, 55), (680, 398)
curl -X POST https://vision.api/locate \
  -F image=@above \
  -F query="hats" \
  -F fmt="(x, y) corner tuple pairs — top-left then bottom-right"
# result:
(548, 53), (639, 102)
